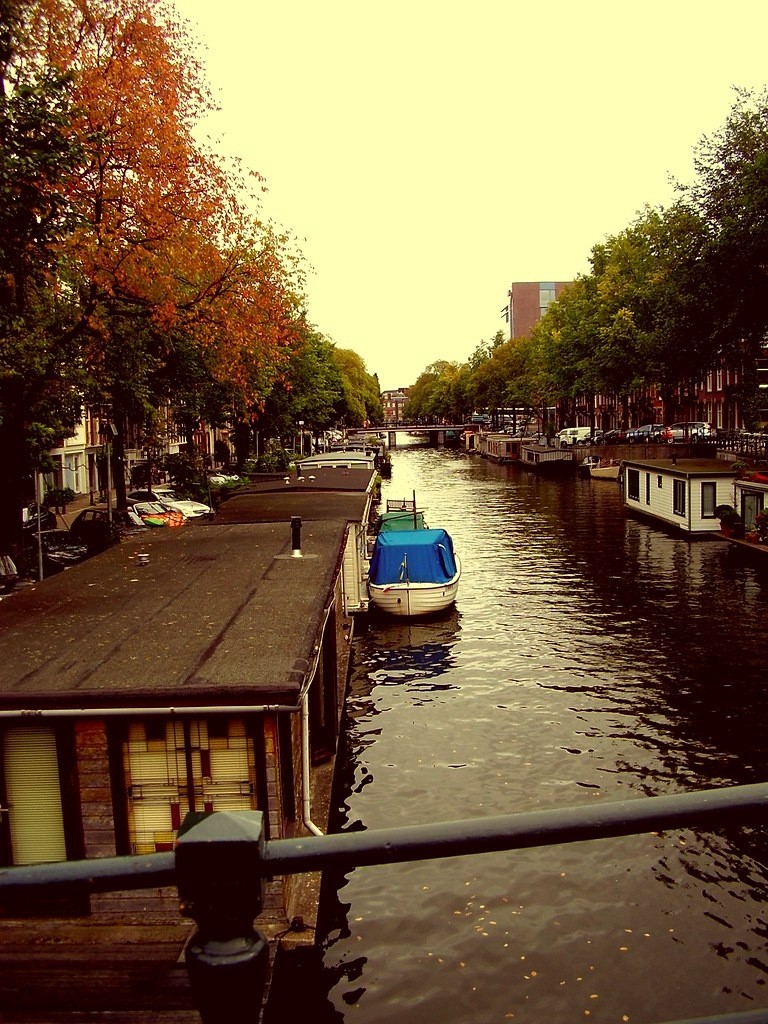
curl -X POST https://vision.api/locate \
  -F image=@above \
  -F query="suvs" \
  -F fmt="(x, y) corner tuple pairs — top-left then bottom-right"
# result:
(667, 421), (717, 443)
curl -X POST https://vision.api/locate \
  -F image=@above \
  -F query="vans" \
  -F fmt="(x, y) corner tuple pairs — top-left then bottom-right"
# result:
(554, 426), (598, 447)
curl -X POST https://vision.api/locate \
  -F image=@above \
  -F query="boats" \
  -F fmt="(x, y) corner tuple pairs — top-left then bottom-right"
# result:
(366, 529), (463, 617)
(378, 498), (430, 532)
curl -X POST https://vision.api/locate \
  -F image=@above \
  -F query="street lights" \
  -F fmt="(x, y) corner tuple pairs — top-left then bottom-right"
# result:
(255, 430), (260, 458)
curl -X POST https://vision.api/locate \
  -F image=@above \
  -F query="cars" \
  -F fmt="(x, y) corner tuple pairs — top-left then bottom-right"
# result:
(203, 467), (239, 485)
(499, 426), (543, 439)
(126, 488), (215, 518)
(125, 501), (187, 528)
(15, 502), (56, 540)
(69, 507), (151, 549)
(585, 422), (675, 447)
